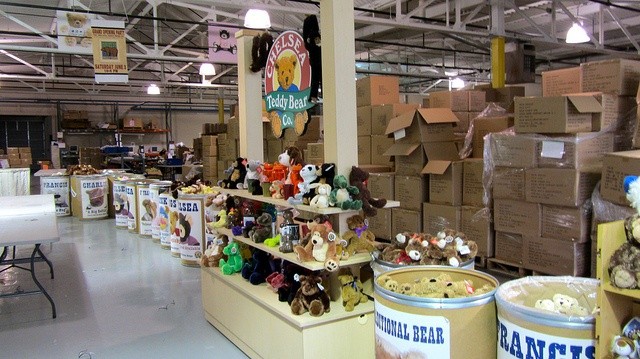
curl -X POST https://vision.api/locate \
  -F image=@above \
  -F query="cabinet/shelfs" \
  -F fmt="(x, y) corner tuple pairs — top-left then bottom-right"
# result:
(201, 189), (400, 359)
(61, 127), (172, 159)
(596, 220), (640, 359)
(506, 42), (535, 83)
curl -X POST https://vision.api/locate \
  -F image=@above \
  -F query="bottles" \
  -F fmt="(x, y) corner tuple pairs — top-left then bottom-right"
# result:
(129, 119), (135, 126)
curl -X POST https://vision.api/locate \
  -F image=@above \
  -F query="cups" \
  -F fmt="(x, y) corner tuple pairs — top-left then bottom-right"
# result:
(42, 165), (49, 170)
(280, 184), (294, 200)
(287, 224), (299, 242)
(247, 179), (255, 192)
(263, 182), (272, 197)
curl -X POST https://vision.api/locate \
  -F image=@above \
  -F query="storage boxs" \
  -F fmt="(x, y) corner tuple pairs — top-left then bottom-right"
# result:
(474, 116), (513, 158)
(453, 111), (469, 132)
(423, 202), (461, 236)
(581, 59), (640, 95)
(383, 143), (460, 176)
(600, 149), (640, 207)
(514, 92), (601, 133)
(495, 231), (523, 264)
(357, 105), (372, 136)
(489, 134), (538, 168)
(356, 76), (399, 105)
(475, 85), (495, 102)
(469, 92), (485, 111)
(149, 121), (158, 128)
(592, 95), (631, 132)
(495, 86), (525, 112)
(430, 91), (468, 111)
(538, 133), (613, 168)
(523, 238), (587, 278)
(395, 176), (426, 210)
(372, 104), (420, 134)
(358, 136), (371, 164)
(358, 164), (389, 173)
(524, 168), (594, 207)
(469, 111), (481, 126)
(541, 206), (590, 243)
(78, 148), (102, 170)
(493, 166), (524, 201)
(371, 134), (394, 165)
(463, 158), (493, 207)
(542, 67), (582, 94)
(193, 104), (240, 185)
(422, 159), (463, 205)
(494, 199), (541, 237)
(0, 147), (33, 167)
(392, 207), (421, 239)
(462, 208), (493, 257)
(121, 117), (143, 127)
(385, 108), (458, 143)
(367, 172), (394, 201)
(107, 122), (117, 128)
(262, 115), (324, 166)
(365, 208), (392, 241)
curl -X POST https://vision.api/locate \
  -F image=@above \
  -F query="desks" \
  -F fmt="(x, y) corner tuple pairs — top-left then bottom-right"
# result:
(182, 165), (202, 181)
(0, 194), (59, 318)
(158, 164), (182, 180)
(0, 168), (30, 196)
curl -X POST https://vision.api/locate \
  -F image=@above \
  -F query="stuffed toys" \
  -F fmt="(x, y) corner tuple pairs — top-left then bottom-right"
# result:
(200, 194), (293, 303)
(342, 216), (376, 259)
(535, 299), (555, 314)
(300, 163), (336, 206)
(310, 183), (335, 211)
(278, 146), (307, 199)
(217, 162), (235, 187)
(293, 214), (335, 259)
(295, 223), (339, 274)
(288, 162), (319, 205)
(330, 175), (363, 210)
(377, 229), (478, 274)
(269, 178), (286, 199)
(237, 160), (263, 190)
(606, 213), (640, 291)
(291, 163), (305, 199)
(351, 165), (387, 217)
(220, 158), (248, 189)
(291, 274), (330, 317)
(338, 266), (369, 312)
(577, 307), (588, 317)
(609, 335), (640, 359)
(257, 162), (288, 198)
(553, 294), (579, 315)
(380, 267), (494, 308)
